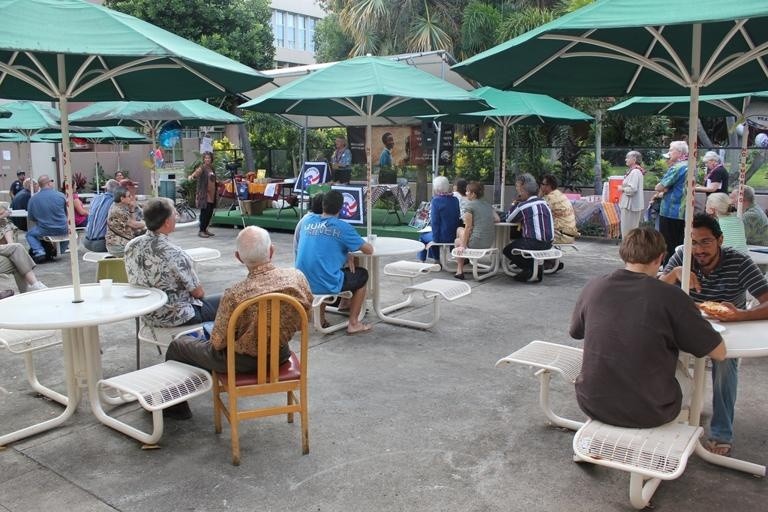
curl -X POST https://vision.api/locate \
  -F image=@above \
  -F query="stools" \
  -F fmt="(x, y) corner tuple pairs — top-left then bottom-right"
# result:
(510, 248), (561, 281)
(384, 258), (443, 301)
(401, 279), (474, 327)
(97, 358), (213, 447)
(94, 257), (127, 283)
(4, 332), (61, 386)
(137, 322), (204, 352)
(573, 417), (703, 508)
(0, 192), (157, 256)
(497, 339), (583, 420)
(453, 248), (498, 281)
(428, 240), (456, 269)
(550, 242), (578, 283)
(307, 292), (354, 333)
(182, 247), (220, 276)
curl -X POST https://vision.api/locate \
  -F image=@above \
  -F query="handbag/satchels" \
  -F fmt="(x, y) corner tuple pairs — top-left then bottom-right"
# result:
(28, 239), (57, 261)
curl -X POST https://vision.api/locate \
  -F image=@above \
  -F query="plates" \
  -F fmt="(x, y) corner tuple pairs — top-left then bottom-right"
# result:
(127, 289), (151, 298)
(707, 322), (726, 332)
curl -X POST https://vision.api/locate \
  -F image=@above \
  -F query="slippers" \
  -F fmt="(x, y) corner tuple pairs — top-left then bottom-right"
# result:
(346, 322), (375, 336)
(337, 305), (369, 314)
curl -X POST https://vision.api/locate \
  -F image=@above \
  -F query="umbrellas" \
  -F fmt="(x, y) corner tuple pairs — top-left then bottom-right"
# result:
(55, 99), (246, 197)
(0, 0), (274, 303)
(448, 0), (768, 298)
(0, 99), (152, 150)
(237, 53), (497, 239)
(606, 88), (768, 218)
(434, 86), (596, 211)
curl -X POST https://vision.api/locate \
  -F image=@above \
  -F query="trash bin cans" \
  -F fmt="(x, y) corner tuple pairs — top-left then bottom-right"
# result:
(159, 181), (175, 203)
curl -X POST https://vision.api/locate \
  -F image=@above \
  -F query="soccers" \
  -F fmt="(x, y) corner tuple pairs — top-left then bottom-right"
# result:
(441, 151), (452, 164)
(755, 133), (767, 148)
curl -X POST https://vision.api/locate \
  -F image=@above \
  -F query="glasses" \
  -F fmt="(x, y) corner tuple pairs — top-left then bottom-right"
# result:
(690, 235), (719, 248)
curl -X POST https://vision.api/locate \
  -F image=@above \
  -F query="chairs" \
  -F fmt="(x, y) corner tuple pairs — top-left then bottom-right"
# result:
(212, 291), (308, 462)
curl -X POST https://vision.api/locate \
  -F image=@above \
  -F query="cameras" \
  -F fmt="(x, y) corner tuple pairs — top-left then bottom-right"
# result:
(223, 157), (243, 169)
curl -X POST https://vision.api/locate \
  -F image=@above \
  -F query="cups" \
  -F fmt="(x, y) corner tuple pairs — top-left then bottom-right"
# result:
(99, 279), (113, 297)
(368, 235), (377, 246)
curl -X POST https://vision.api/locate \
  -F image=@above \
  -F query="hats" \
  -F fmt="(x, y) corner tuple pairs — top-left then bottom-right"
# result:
(119, 178), (140, 188)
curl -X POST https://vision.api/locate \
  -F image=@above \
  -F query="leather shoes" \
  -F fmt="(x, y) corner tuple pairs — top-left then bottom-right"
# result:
(198, 231), (215, 238)
(0, 289), (15, 299)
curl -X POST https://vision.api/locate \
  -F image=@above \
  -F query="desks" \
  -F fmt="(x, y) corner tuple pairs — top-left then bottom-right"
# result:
(348, 236), (425, 316)
(213, 183), (407, 226)
(674, 312), (767, 456)
(0, 283), (168, 449)
(492, 223), (518, 276)
(658, 243), (768, 445)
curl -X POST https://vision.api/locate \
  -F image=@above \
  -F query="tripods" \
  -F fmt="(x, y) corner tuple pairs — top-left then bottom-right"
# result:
(208, 170), (249, 229)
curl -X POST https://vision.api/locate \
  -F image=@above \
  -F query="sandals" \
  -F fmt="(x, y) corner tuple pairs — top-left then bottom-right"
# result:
(705, 438), (733, 460)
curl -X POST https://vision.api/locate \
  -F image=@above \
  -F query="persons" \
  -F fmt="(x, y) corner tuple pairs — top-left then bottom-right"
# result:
(150, 225), (315, 421)
(292, 192), (326, 264)
(330, 133), (353, 184)
(296, 190), (374, 334)
(416, 172), (577, 282)
(124, 197), (225, 327)
(569, 227), (727, 463)
(659, 212), (768, 455)
(379, 133), (397, 183)
(617, 140), (768, 247)
(0, 169), (148, 300)
(188, 151), (216, 238)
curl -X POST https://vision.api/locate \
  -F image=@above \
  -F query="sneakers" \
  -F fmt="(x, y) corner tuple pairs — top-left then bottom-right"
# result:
(573, 436), (604, 463)
(26, 281), (49, 292)
(455, 258), (563, 284)
(34, 256), (46, 264)
(162, 401), (192, 419)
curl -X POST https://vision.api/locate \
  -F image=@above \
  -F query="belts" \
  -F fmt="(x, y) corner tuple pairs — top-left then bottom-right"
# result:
(85, 236), (105, 241)
